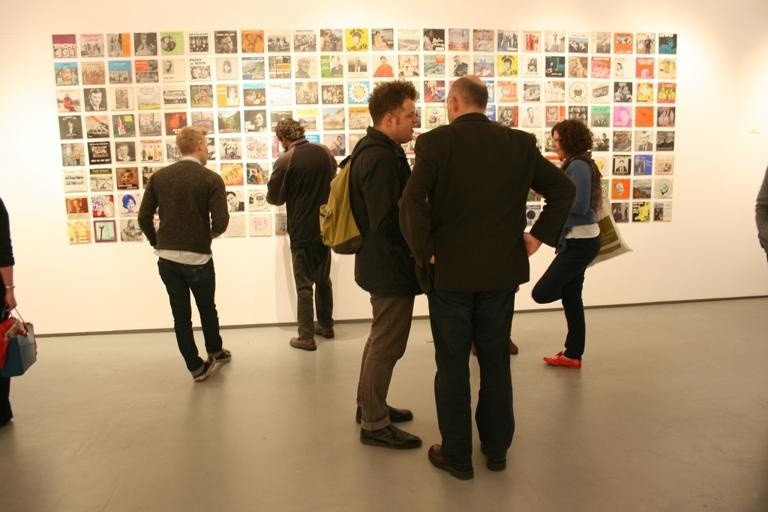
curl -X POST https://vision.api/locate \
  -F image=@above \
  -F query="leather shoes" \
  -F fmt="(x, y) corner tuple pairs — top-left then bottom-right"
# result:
(481, 443), (508, 471)
(356, 406), (412, 424)
(543, 356), (581, 368)
(314, 322), (334, 338)
(361, 424), (421, 449)
(510, 340), (519, 354)
(428, 444), (473, 479)
(290, 337), (316, 351)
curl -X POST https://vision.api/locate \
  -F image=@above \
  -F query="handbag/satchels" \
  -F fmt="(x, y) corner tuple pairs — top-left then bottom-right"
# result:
(0, 304), (36, 377)
(588, 198), (632, 267)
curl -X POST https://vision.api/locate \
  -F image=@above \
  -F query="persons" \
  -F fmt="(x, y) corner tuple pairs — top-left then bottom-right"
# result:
(532, 119), (606, 368)
(267, 117), (338, 351)
(755, 168), (767, 261)
(50, 26), (345, 246)
(345, 24), (678, 225)
(0, 197), (16, 431)
(397, 74), (576, 481)
(138, 128), (232, 383)
(344, 77), (426, 450)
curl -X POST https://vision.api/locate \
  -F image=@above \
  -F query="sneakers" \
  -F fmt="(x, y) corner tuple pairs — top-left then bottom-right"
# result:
(215, 350), (230, 361)
(194, 356), (215, 381)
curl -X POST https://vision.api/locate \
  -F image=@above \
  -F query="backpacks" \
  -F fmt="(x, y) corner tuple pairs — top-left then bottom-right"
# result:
(319, 136), (393, 254)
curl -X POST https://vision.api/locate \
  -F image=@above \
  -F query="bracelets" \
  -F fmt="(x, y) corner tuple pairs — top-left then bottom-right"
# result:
(4, 285), (16, 290)
